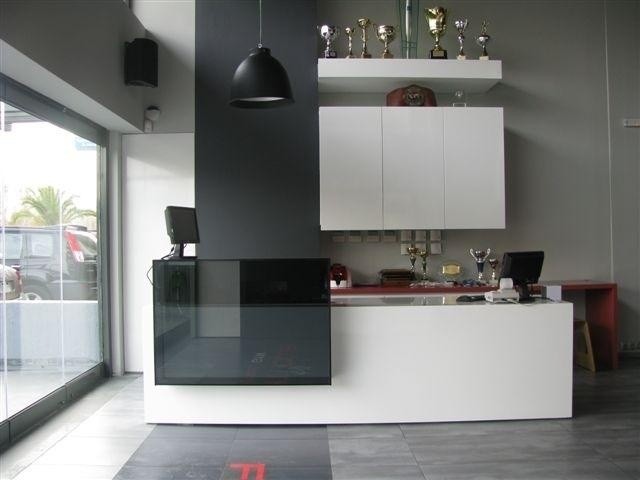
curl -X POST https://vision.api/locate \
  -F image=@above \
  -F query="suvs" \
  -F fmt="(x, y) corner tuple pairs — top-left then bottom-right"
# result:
(0, 224), (97, 300)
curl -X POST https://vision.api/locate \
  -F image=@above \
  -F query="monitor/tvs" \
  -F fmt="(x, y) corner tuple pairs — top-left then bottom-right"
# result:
(164, 205), (200, 259)
(498, 251), (544, 302)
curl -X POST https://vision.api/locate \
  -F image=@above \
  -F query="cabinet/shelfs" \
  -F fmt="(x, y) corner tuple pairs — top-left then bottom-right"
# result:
(318, 106), (506, 231)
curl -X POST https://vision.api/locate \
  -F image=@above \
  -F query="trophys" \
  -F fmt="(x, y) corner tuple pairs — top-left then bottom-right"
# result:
(423, 6), (450, 60)
(342, 26), (358, 59)
(489, 257), (499, 281)
(406, 246), (419, 273)
(468, 247), (491, 280)
(316, 23), (342, 59)
(371, 21), (401, 59)
(419, 249), (430, 280)
(357, 17), (372, 59)
(475, 18), (492, 60)
(454, 18), (471, 59)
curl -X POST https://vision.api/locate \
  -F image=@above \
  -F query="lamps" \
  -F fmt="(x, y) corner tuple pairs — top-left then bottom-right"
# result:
(228, 0), (295, 110)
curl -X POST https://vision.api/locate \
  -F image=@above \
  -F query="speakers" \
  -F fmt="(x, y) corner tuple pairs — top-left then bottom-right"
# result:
(127, 38), (159, 87)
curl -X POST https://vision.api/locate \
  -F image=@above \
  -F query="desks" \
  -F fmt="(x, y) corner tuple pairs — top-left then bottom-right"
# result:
(330, 279), (619, 371)
